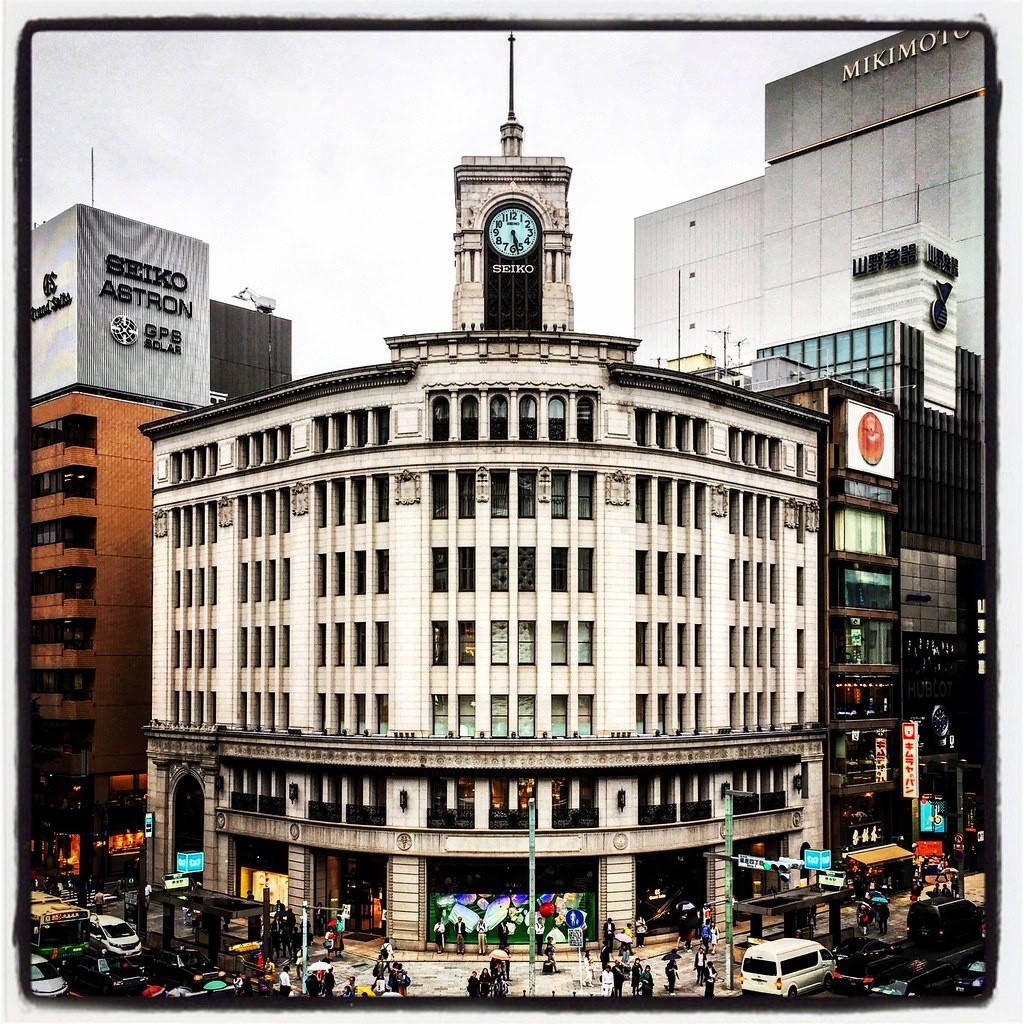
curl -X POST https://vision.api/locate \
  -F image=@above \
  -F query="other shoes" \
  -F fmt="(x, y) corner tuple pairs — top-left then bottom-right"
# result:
(663, 985), (669, 990)
(687, 949), (692, 952)
(684, 946), (687, 951)
(339, 954), (343, 958)
(618, 954), (623, 956)
(638, 945), (641, 947)
(695, 981), (699, 984)
(506, 979), (513, 981)
(699, 984), (703, 986)
(642, 945), (644, 947)
(673, 988), (680, 991)
(631, 952), (636, 956)
(335, 955), (339, 958)
(582, 981), (586, 986)
(711, 950), (716, 954)
(587, 983), (594, 987)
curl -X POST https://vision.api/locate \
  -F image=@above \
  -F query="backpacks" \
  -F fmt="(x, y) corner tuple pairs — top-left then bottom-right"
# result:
(306, 979), (313, 991)
(373, 961), (380, 977)
(381, 944), (390, 960)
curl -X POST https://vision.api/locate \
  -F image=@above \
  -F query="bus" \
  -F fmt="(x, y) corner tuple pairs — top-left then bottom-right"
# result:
(28, 889), (99, 964)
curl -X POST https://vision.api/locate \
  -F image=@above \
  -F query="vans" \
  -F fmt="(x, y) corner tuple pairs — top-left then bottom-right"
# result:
(740, 937), (836, 1002)
(906, 895), (985, 950)
(80, 915), (142, 957)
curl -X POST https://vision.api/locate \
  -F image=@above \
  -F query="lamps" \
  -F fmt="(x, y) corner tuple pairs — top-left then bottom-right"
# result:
(610, 723), (822, 739)
(543, 731), (547, 738)
(394, 732), (415, 738)
(342, 730), (348, 735)
(721, 781), (730, 800)
(323, 729), (327, 736)
(289, 782), (299, 804)
(794, 773), (802, 794)
(573, 732), (579, 738)
(447, 731), (453, 737)
(363, 730), (369, 736)
(617, 789), (626, 814)
(154, 718), (227, 733)
(511, 732), (516, 738)
(480, 731), (484, 738)
(400, 788), (408, 813)
(216, 775), (225, 792)
(286, 728), (302, 737)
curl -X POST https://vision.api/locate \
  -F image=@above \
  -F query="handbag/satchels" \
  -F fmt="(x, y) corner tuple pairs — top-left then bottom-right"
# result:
(376, 976), (386, 992)
(401, 971), (410, 987)
(323, 939), (332, 948)
(295, 956), (303, 965)
(542, 958), (553, 973)
(544, 945), (551, 956)
(714, 927), (720, 936)
(643, 925), (647, 931)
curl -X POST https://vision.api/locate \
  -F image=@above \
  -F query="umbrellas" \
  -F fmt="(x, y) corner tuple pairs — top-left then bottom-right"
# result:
(326, 919), (338, 927)
(872, 897), (889, 904)
(614, 933), (633, 945)
(940, 867), (959, 876)
(857, 901), (872, 911)
(661, 953), (682, 961)
(675, 901), (696, 911)
(203, 981), (227, 990)
(141, 986), (165, 997)
(870, 890), (885, 898)
(307, 960), (332, 981)
(489, 949), (509, 961)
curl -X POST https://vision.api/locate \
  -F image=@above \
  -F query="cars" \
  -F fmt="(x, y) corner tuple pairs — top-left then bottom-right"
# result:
(139, 945), (228, 994)
(28, 953), (69, 998)
(55, 950), (150, 999)
(826, 936), (988, 999)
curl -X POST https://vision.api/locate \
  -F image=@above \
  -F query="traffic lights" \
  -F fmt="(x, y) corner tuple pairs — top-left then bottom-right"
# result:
(764, 861), (790, 882)
(336, 914), (345, 933)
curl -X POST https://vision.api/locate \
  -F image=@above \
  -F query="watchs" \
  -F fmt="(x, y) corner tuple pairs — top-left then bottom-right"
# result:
(932, 705), (952, 744)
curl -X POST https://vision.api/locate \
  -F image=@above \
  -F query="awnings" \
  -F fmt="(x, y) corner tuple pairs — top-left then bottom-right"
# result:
(844, 843), (917, 867)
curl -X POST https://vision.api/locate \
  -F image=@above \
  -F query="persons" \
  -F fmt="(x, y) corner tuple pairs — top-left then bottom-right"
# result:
(18, 859), (960, 1007)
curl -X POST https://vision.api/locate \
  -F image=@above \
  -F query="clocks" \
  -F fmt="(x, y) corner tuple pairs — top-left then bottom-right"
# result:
(485, 205), (542, 260)
